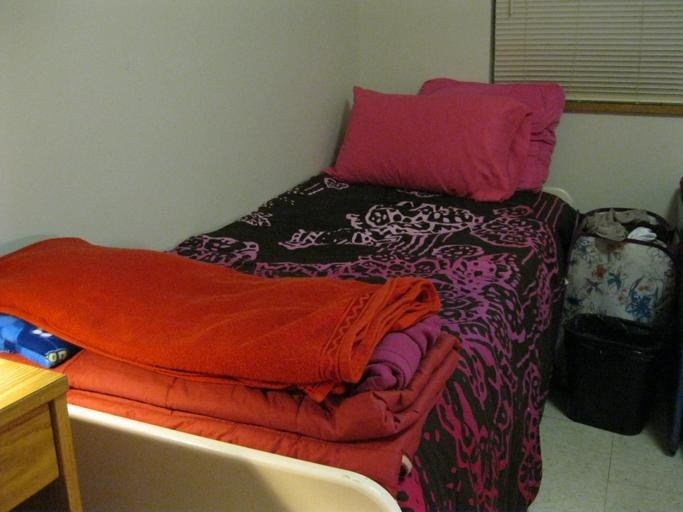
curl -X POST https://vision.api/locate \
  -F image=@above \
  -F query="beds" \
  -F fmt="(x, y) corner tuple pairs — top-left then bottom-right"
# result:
(11, 174), (586, 512)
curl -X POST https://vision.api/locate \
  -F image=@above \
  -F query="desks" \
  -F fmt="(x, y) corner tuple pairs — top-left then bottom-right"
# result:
(0, 359), (80, 511)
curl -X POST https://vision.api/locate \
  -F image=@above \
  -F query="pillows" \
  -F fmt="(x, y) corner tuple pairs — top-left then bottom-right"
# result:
(323, 84), (532, 203)
(419, 77), (564, 193)
